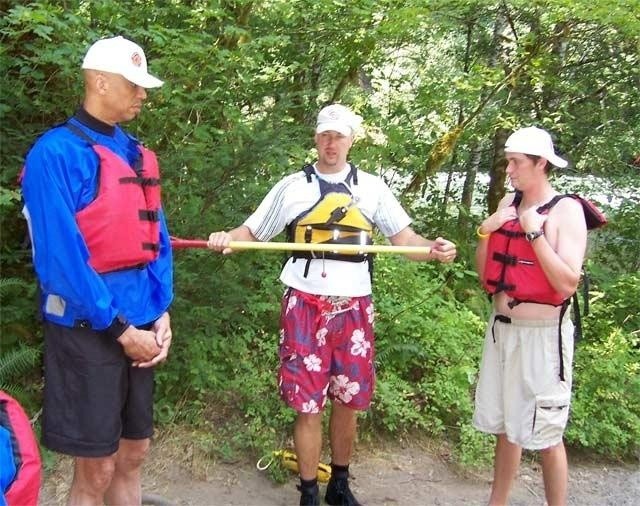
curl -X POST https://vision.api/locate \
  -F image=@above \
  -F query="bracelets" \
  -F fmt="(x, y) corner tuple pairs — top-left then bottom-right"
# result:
(476, 225), (491, 238)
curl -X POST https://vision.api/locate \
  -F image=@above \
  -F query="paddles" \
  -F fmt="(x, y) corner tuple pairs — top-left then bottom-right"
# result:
(170, 235), (439, 255)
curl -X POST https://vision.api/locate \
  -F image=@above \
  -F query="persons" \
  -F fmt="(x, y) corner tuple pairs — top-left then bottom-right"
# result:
(475, 127), (588, 506)
(208, 103), (457, 506)
(23, 36), (174, 506)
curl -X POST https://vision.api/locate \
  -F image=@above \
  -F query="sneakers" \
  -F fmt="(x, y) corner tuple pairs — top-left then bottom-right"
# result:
(295, 471), (363, 506)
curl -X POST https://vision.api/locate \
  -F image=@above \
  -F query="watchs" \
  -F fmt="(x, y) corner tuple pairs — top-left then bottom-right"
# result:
(525, 230), (546, 241)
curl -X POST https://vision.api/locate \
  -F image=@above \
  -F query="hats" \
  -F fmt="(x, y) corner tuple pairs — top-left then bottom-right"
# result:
(81, 35), (165, 89)
(504, 126), (568, 169)
(316, 103), (357, 137)
